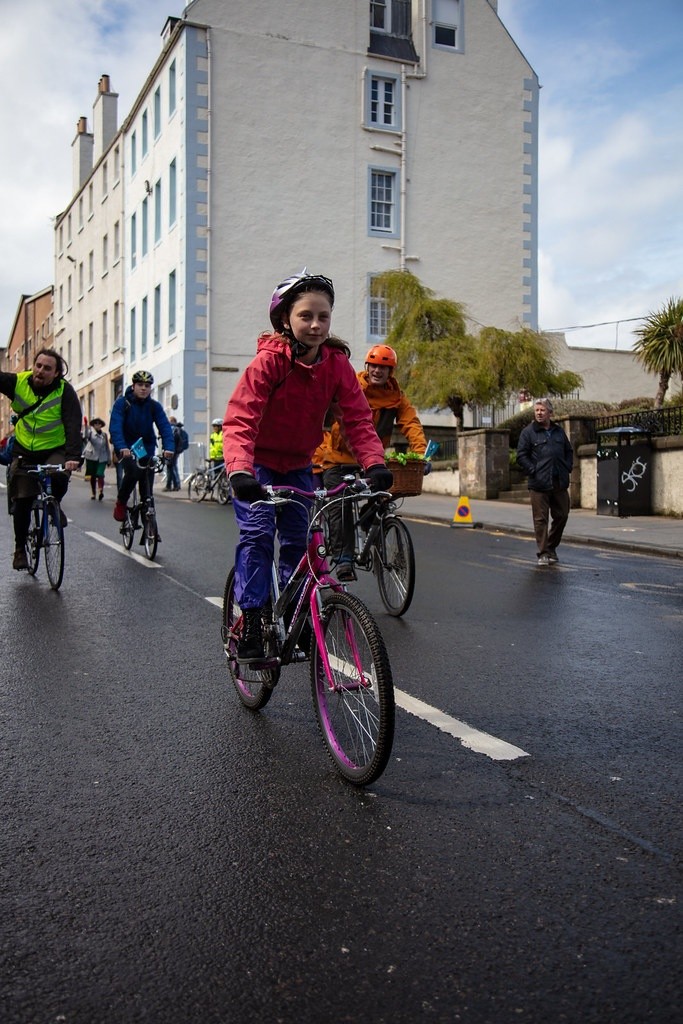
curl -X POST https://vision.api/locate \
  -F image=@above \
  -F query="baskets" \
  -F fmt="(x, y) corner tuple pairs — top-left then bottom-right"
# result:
(386, 457), (427, 496)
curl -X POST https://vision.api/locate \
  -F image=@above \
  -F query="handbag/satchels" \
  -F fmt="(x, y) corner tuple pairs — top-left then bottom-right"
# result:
(0, 435), (16, 467)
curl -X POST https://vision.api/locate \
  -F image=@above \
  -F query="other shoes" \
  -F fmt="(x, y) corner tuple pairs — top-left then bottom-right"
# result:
(538, 554), (549, 565)
(172, 488), (179, 491)
(113, 498), (128, 522)
(549, 550), (559, 562)
(13, 547), (28, 569)
(47, 501), (68, 527)
(336, 562), (352, 579)
(162, 488), (172, 492)
(157, 534), (162, 542)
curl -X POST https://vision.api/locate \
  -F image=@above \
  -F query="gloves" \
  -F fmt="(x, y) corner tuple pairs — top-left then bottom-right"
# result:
(364, 464), (394, 493)
(230, 472), (271, 503)
(424, 460), (432, 476)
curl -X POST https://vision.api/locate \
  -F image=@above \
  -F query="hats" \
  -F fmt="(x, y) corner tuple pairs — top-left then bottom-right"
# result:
(89, 418), (106, 427)
(534, 398), (556, 417)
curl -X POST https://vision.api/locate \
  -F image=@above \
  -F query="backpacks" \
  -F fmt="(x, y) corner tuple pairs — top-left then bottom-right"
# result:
(173, 423), (189, 454)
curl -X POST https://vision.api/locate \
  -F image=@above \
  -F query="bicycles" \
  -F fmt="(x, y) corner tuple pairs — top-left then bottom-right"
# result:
(16, 454), (77, 592)
(118, 452), (171, 562)
(184, 456), (235, 505)
(312, 457), (428, 617)
(220, 463), (396, 787)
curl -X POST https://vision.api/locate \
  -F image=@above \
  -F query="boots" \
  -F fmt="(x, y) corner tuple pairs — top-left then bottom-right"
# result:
(282, 609), (311, 658)
(236, 607), (266, 665)
(91, 489), (96, 499)
(98, 488), (103, 501)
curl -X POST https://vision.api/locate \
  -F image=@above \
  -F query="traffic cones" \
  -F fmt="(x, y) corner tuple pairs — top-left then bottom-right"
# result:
(450, 495), (474, 528)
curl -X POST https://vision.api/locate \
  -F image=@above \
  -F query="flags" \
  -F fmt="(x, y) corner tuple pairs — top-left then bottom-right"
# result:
(425, 441), (440, 457)
(132, 438), (147, 458)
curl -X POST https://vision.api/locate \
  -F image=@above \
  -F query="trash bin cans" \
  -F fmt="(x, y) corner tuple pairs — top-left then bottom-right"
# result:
(598, 427), (655, 518)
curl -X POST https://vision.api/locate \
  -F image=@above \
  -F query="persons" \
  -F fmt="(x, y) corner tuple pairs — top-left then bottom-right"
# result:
(109, 370), (174, 546)
(311, 432), (330, 491)
(210, 418), (232, 503)
(82, 415), (110, 500)
(322, 344), (430, 579)
(162, 417), (180, 491)
(0, 349), (83, 570)
(223, 266), (393, 663)
(516, 398), (573, 565)
(112, 450), (123, 489)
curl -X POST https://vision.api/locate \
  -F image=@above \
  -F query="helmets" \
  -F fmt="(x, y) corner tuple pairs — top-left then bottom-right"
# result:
(211, 418), (225, 426)
(365, 345), (398, 378)
(269, 265), (335, 336)
(132, 371), (155, 385)
